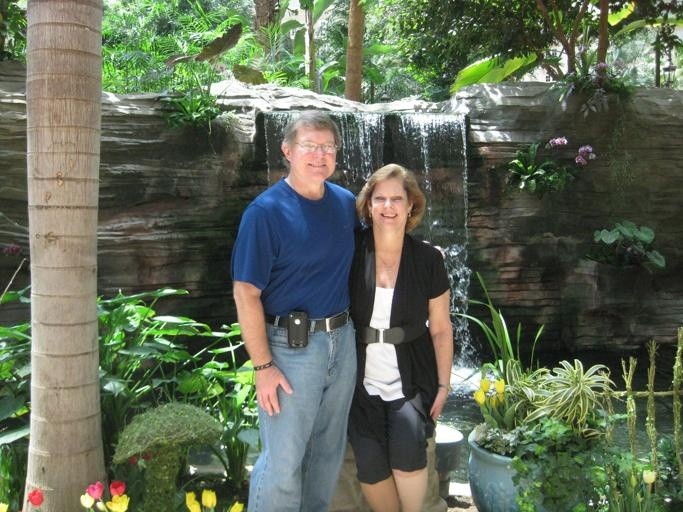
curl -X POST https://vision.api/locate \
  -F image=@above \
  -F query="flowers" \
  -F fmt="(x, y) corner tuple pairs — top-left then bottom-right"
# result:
(449, 271), (618, 461)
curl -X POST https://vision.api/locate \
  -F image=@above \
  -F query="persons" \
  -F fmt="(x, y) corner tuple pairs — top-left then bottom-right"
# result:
(348, 160), (459, 512)
(228, 108), (448, 512)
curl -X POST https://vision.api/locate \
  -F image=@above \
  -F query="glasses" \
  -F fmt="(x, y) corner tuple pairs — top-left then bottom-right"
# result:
(294, 141), (337, 153)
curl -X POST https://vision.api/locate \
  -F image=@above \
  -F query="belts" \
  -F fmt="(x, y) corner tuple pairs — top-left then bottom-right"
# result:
(264, 310), (348, 333)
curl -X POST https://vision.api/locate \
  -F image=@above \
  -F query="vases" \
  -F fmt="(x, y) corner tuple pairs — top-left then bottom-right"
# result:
(467, 423), (614, 512)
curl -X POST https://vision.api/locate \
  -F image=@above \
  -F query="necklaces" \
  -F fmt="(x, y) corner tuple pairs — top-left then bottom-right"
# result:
(376, 252), (397, 270)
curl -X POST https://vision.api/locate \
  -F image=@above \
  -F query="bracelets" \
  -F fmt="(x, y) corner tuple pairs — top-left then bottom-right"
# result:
(252, 360), (274, 371)
(436, 383), (451, 390)
